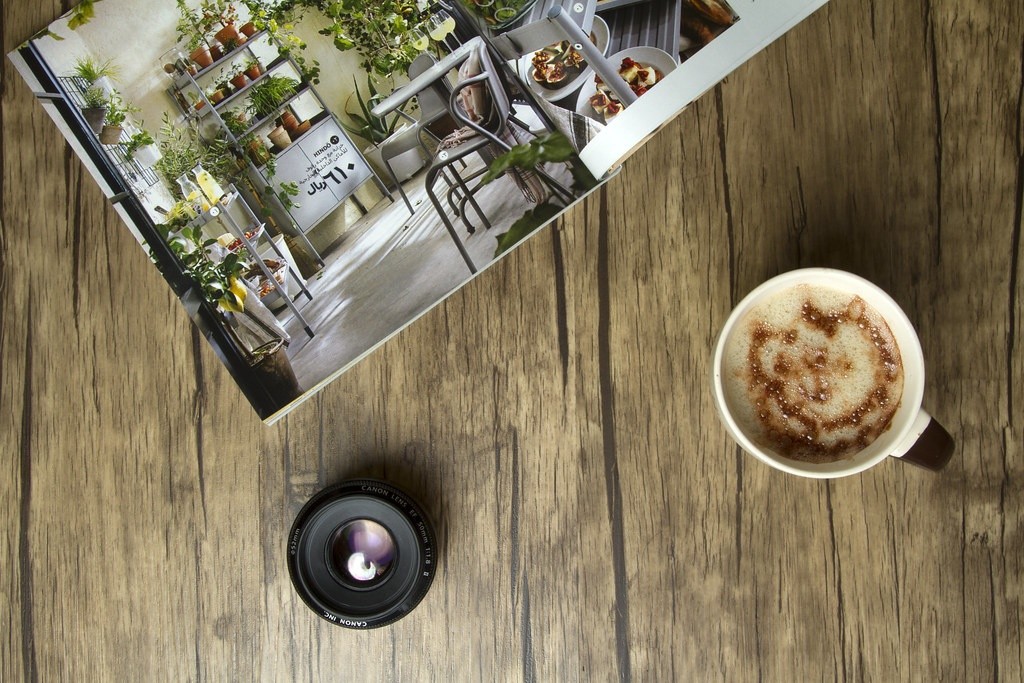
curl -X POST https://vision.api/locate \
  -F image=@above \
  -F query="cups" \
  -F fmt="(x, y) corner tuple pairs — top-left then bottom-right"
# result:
(712, 265), (956, 481)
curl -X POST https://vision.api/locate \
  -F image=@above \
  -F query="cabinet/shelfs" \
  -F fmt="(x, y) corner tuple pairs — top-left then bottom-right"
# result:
(169, 182), (315, 348)
(164, 25), (396, 267)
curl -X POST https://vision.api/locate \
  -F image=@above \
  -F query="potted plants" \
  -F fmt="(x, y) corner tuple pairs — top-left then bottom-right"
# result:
(200, 0), (241, 49)
(225, 61), (247, 90)
(336, 72), (423, 189)
(252, 55), (267, 75)
(141, 216), (306, 419)
(176, 18), (213, 69)
(187, 88), (206, 110)
(72, 54), (142, 144)
(211, 68), (233, 97)
(151, 120), (308, 313)
(176, 0), (223, 63)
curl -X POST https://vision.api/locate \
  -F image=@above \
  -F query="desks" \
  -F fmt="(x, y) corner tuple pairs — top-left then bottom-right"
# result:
(369, 35), (553, 234)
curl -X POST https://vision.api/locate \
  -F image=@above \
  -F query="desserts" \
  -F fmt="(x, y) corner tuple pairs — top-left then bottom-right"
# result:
(531, 41), (585, 90)
(589, 58), (655, 124)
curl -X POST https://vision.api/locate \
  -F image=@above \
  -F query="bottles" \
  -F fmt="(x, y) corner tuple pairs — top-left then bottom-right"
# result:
(175, 165), (228, 215)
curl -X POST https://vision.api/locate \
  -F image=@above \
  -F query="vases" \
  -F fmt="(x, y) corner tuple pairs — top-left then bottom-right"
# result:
(242, 135), (270, 166)
(239, 21), (258, 38)
(243, 65), (261, 81)
(270, 130), (293, 151)
(267, 125), (285, 140)
(208, 88), (224, 102)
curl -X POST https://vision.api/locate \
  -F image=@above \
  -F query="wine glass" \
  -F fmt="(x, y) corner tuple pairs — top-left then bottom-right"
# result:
(409, 9), (469, 70)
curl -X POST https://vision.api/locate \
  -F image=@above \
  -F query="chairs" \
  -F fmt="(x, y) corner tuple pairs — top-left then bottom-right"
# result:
(381, 40), (579, 273)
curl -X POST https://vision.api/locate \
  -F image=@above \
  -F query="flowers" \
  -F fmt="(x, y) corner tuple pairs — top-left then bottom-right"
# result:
(242, 59), (258, 70)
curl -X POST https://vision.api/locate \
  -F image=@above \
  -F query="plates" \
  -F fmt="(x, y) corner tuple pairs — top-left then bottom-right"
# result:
(527, 14), (679, 126)
